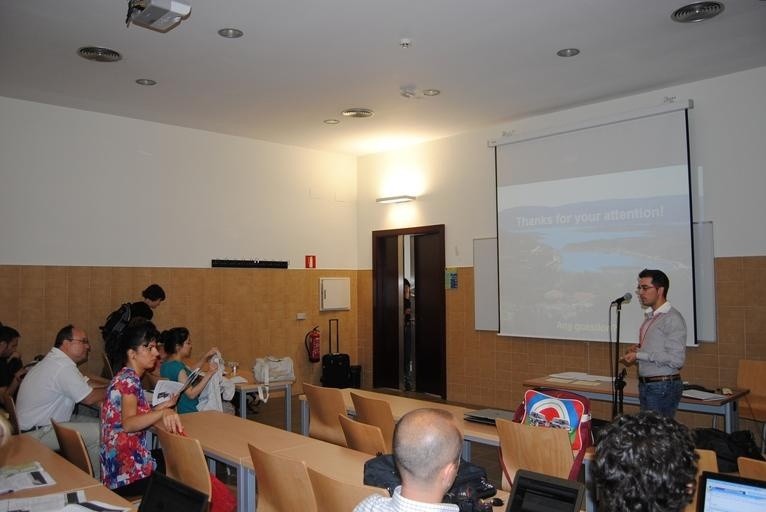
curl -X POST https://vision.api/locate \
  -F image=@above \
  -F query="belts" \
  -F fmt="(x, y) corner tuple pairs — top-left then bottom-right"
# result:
(638, 374), (680, 382)
(21, 425), (42, 433)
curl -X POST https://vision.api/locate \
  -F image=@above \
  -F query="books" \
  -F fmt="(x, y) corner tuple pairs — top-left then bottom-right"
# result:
(150, 365), (202, 407)
(680, 388), (727, 401)
(0, 458), (128, 511)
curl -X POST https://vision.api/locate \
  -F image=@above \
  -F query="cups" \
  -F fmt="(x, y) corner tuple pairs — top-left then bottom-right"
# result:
(305, 255), (316, 268)
(228, 362), (240, 375)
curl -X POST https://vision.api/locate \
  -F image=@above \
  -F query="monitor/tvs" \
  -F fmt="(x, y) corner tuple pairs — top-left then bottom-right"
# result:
(695, 471), (766, 512)
(505, 469), (586, 512)
(137, 470), (209, 512)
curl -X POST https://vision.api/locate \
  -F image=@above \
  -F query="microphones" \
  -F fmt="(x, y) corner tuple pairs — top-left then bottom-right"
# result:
(612, 293), (633, 305)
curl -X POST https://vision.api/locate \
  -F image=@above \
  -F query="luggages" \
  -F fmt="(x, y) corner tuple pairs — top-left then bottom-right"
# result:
(321, 318), (350, 388)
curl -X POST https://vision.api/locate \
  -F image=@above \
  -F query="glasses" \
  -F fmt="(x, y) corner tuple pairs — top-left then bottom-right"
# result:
(68, 338), (89, 343)
(637, 286), (654, 290)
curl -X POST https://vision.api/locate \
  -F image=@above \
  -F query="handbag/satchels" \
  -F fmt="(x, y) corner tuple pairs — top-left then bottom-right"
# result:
(102, 302), (131, 341)
(363, 454), (496, 503)
(210, 472), (237, 511)
(253, 357), (294, 382)
(513, 388), (593, 449)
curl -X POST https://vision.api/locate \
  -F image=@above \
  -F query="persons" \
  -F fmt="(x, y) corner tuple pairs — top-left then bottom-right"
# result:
(158, 328), (237, 416)
(95, 316), (184, 499)
(101, 281), (168, 379)
(403, 277), (414, 386)
(350, 408), (466, 512)
(618, 266), (695, 424)
(585, 410), (701, 511)
(17, 324), (109, 484)
(0, 417), (13, 466)
(1, 325), (26, 429)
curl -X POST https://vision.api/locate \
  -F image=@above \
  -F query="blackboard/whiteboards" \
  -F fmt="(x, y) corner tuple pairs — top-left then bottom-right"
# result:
(473, 221), (716, 343)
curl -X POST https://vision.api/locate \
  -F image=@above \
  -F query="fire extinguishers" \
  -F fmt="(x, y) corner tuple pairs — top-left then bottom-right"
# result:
(305, 326), (320, 362)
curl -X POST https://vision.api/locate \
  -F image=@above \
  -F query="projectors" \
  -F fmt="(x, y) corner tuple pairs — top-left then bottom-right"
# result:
(125, 0), (192, 33)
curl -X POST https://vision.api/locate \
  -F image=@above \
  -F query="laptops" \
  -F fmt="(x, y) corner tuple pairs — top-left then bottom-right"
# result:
(464, 408), (515, 426)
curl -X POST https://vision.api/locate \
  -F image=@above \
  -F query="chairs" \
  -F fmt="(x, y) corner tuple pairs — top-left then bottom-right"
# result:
(0, 348), (766, 512)
